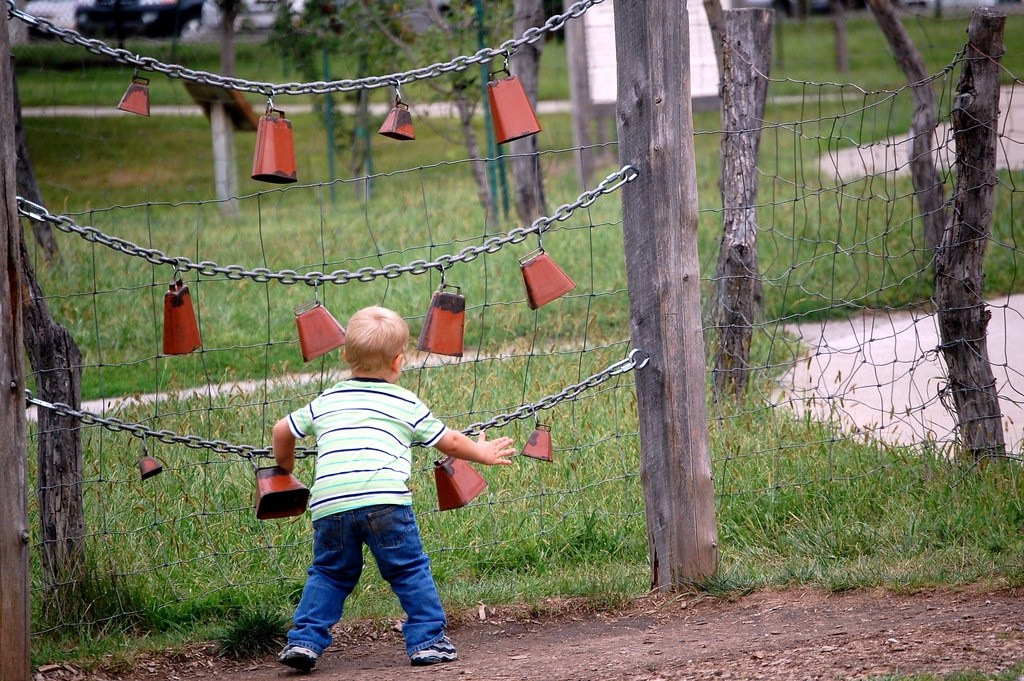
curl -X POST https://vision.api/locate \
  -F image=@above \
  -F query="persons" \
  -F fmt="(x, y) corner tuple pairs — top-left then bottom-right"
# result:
(272, 306), (516, 670)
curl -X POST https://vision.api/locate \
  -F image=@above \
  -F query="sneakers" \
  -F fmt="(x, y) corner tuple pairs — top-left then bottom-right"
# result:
(411, 635), (457, 665)
(277, 644), (317, 673)
